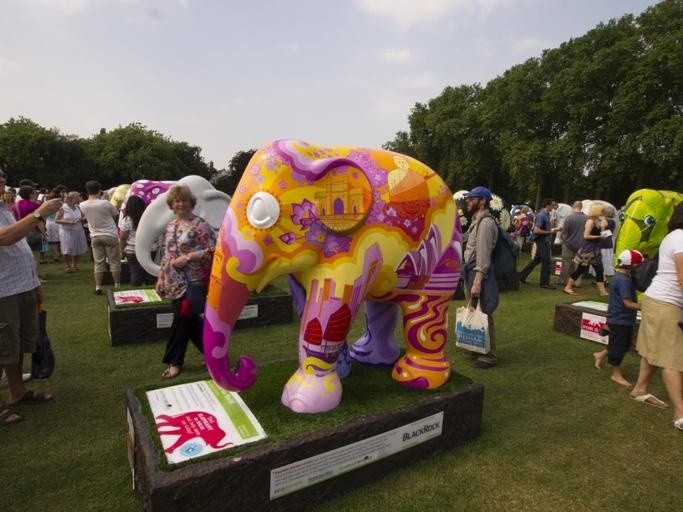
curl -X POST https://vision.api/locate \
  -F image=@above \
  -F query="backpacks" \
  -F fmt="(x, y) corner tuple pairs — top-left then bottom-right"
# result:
(476, 216), (520, 281)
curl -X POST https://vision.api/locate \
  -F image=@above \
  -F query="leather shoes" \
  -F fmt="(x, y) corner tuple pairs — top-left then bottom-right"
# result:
(541, 285), (556, 288)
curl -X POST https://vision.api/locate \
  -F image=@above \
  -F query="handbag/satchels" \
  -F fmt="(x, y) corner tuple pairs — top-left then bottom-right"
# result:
(31, 311), (54, 378)
(186, 282), (208, 314)
(456, 298), (489, 354)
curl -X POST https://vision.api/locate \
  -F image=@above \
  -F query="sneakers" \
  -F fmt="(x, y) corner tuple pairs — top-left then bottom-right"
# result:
(463, 351), (475, 357)
(476, 362), (497, 368)
(162, 366), (178, 377)
(95, 290), (102, 294)
(0, 373), (31, 388)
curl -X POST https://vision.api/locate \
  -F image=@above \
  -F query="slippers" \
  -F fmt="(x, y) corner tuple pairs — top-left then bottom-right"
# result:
(7, 390), (53, 404)
(0, 406), (22, 423)
(563, 290), (577, 296)
(627, 393), (668, 410)
(674, 418), (683, 430)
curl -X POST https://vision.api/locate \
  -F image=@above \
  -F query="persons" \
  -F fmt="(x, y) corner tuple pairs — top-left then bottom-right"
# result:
(0, 179), (96, 283)
(119, 195), (160, 287)
(592, 248), (645, 387)
(460, 186), (498, 368)
(0, 366), (32, 388)
(0, 168), (63, 424)
(506, 198), (624, 296)
(77, 180), (122, 295)
(154, 185), (216, 378)
(626, 200), (683, 432)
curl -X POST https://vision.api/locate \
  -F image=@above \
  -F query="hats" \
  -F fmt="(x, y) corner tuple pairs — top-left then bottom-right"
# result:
(469, 187), (492, 200)
(617, 250), (643, 267)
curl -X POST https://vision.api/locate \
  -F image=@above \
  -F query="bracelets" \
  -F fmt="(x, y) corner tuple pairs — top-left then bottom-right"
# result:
(185, 255), (189, 262)
